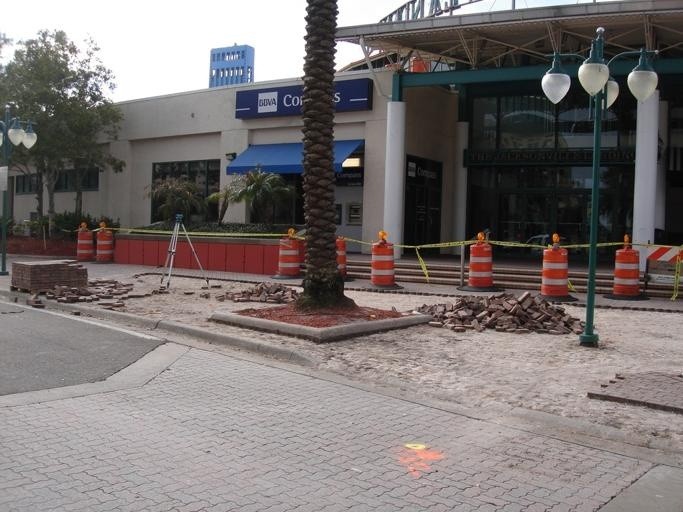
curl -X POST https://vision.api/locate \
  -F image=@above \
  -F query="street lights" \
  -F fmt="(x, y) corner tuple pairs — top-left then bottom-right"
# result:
(540, 26), (658, 349)
(0, 105), (38, 276)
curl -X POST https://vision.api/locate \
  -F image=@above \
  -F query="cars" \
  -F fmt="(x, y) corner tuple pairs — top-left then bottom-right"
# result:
(526, 234), (568, 246)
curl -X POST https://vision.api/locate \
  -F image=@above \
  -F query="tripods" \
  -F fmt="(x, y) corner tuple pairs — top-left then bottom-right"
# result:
(159, 223), (208, 287)
